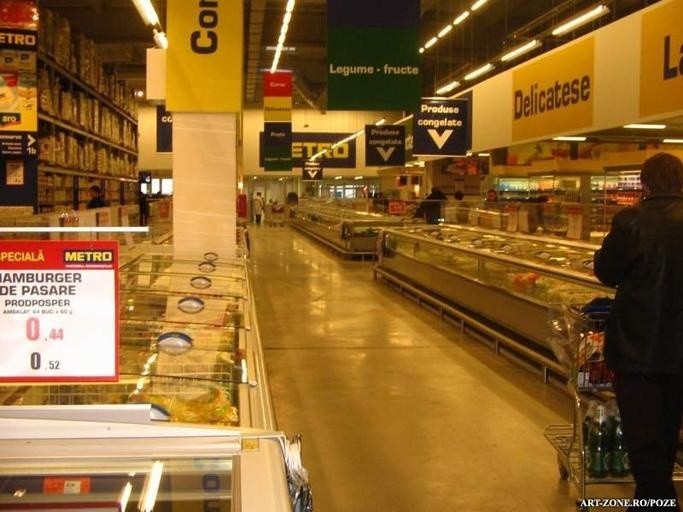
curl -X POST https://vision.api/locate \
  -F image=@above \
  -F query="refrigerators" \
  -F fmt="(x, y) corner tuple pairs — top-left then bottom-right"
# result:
(0, 249), (300, 511)
(286, 200), (615, 360)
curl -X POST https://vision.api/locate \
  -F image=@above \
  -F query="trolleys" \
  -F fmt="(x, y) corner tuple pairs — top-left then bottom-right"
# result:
(539, 282), (682, 512)
(263, 201), (285, 228)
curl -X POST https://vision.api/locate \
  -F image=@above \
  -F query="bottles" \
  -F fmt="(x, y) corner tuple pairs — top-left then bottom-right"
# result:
(581, 394), (634, 478)
(592, 172), (643, 233)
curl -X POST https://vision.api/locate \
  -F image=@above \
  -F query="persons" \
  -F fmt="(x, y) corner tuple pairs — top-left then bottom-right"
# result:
(86, 185), (105, 209)
(367, 185), (498, 225)
(593, 154), (683, 512)
(253, 192), (266, 223)
(135, 191), (150, 226)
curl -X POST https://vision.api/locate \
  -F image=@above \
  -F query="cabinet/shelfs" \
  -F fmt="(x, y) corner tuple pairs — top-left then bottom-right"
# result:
(32, 53), (140, 215)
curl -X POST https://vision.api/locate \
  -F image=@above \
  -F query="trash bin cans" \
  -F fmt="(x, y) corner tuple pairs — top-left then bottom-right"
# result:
(159, 201), (169, 217)
